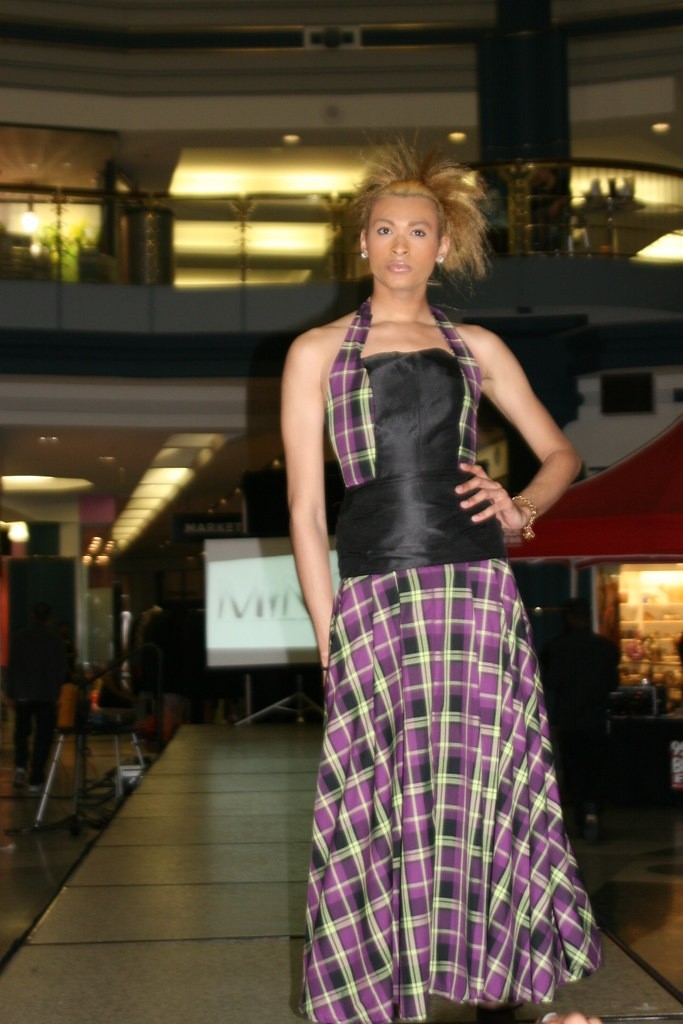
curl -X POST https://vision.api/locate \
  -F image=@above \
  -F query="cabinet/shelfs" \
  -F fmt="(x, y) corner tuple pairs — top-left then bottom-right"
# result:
(619, 591), (683, 681)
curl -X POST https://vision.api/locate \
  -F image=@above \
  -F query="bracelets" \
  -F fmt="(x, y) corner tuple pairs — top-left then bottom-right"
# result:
(511, 496), (537, 541)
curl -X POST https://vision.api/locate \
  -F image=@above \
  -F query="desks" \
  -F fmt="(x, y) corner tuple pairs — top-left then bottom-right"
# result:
(31, 726), (147, 831)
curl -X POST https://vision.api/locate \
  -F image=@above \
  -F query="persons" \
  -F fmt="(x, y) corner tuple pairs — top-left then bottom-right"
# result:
(56, 620), (78, 667)
(277, 144), (603, 1024)
(3, 603), (66, 793)
(540, 594), (623, 839)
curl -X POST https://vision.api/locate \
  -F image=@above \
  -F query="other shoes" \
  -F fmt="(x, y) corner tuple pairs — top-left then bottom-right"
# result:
(581, 814), (599, 844)
(14, 767), (25, 789)
(29, 781), (45, 795)
